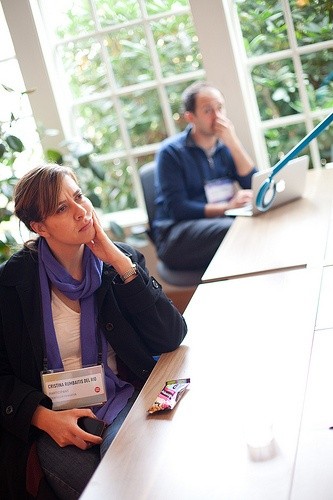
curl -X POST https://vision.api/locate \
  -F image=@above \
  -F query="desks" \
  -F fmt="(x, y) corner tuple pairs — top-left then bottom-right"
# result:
(198, 166), (331, 284)
(76, 269), (331, 497)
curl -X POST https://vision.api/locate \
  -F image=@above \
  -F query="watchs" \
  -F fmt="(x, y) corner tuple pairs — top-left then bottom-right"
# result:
(120, 263), (138, 282)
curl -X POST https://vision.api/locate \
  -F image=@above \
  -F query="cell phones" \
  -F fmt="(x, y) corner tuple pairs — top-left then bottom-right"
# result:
(77, 416), (106, 438)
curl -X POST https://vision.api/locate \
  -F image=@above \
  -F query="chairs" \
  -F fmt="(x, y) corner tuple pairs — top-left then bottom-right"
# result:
(137, 162), (208, 286)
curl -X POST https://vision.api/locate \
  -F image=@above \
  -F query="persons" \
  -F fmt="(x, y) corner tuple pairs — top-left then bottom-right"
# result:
(150, 80), (260, 271)
(1, 163), (190, 480)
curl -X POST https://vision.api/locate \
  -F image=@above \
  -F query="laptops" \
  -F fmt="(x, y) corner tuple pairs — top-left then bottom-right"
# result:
(224, 155), (309, 216)
